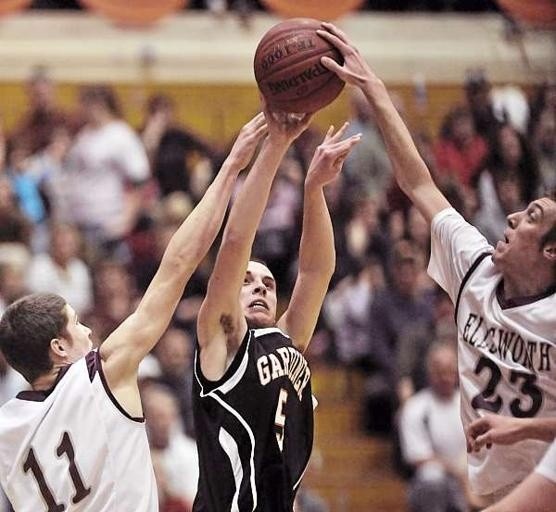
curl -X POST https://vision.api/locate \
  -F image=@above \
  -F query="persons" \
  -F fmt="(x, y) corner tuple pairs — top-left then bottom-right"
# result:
(1, 62), (556, 512)
(315, 21), (556, 512)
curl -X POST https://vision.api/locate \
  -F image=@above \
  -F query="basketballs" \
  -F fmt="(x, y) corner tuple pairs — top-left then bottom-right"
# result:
(251, 16), (347, 115)
